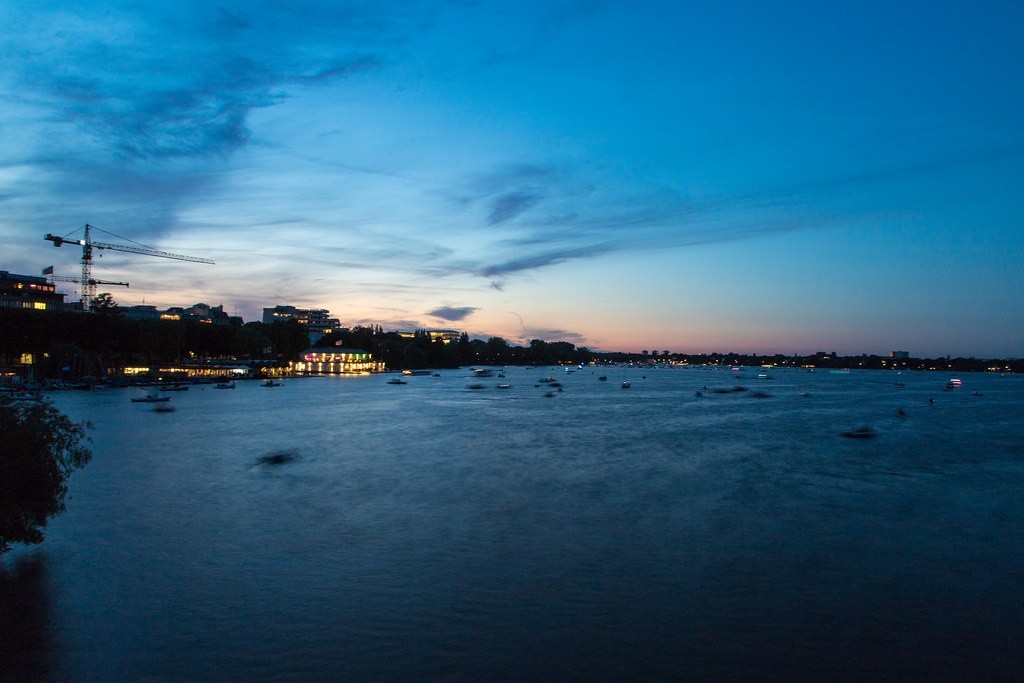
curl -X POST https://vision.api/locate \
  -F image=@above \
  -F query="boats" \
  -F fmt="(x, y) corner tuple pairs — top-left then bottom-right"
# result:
(39, 357), (560, 411)
(131, 395), (171, 402)
(5, 392), (49, 400)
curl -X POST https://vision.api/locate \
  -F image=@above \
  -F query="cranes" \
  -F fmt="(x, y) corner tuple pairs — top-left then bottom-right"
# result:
(43, 223), (216, 314)
